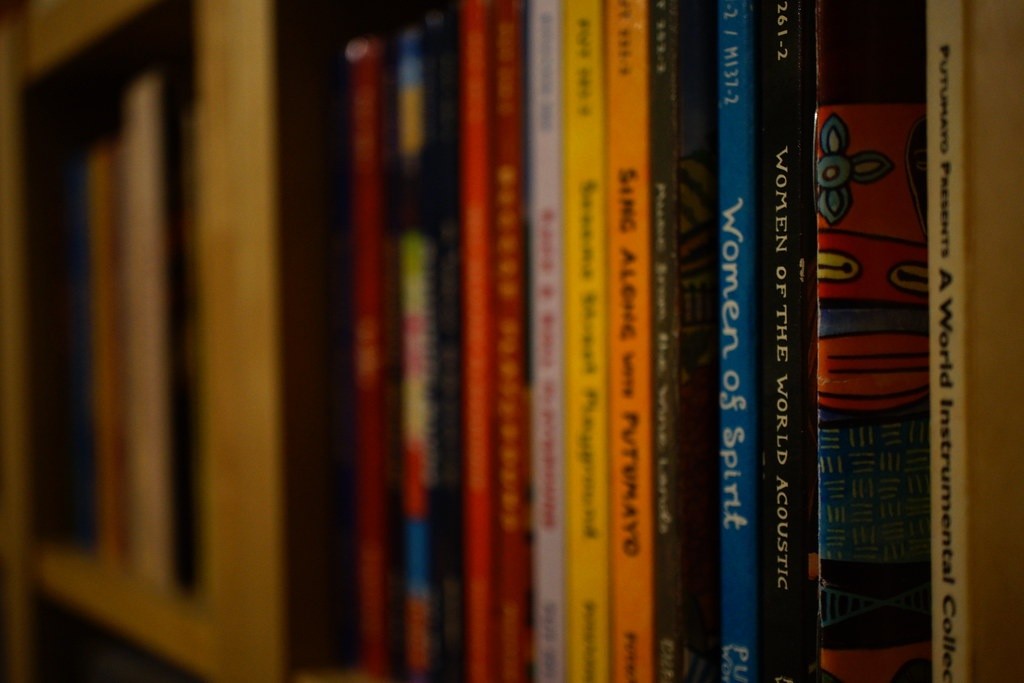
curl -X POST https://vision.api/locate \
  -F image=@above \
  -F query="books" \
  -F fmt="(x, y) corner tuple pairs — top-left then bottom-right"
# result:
(21, 0), (1023, 683)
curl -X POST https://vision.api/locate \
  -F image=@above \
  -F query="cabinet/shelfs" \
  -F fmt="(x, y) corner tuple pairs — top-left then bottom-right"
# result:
(0, 2), (331, 683)
